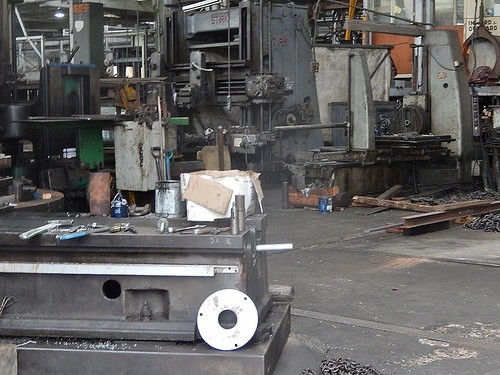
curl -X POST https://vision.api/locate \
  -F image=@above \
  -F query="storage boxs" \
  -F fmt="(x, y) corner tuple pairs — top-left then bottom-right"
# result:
(180, 170), (264, 223)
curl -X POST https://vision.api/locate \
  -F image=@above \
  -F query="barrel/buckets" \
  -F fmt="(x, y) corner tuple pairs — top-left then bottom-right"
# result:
(317, 196), (332, 214)
(155, 180), (186, 219)
